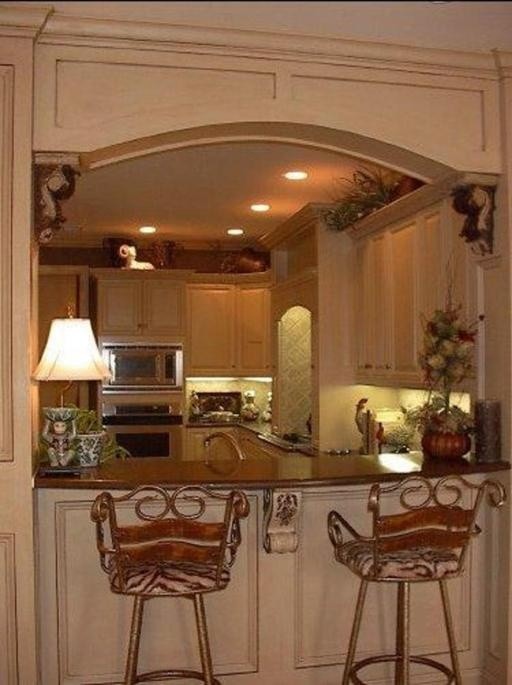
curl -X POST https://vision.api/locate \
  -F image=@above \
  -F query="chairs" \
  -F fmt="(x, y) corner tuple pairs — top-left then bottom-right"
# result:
(90, 482), (251, 684)
(327, 470), (508, 684)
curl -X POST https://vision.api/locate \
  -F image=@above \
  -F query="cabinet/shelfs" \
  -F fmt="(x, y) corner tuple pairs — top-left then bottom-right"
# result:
(184, 268), (236, 378)
(343, 176), (485, 394)
(90, 268), (198, 335)
(237, 268), (274, 377)
(186, 423), (237, 460)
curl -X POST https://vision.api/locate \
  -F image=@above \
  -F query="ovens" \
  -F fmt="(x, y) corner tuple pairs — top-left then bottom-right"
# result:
(99, 414), (183, 460)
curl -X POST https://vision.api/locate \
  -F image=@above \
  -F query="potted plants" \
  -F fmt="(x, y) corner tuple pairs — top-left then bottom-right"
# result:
(66, 403), (107, 467)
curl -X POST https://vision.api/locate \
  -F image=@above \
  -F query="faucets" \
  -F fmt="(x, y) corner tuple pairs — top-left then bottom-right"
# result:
(203, 432), (244, 459)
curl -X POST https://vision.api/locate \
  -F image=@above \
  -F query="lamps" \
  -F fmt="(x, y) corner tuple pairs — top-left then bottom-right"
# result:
(30, 297), (114, 478)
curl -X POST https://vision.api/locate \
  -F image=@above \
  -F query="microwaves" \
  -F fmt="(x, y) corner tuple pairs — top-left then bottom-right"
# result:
(100, 342), (184, 395)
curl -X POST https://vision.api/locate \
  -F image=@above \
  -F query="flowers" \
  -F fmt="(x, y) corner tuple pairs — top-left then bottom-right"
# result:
(398, 304), (485, 435)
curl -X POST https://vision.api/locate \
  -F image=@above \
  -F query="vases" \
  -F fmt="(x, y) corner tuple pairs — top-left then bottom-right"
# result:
(421, 430), (471, 459)
(237, 246), (266, 272)
